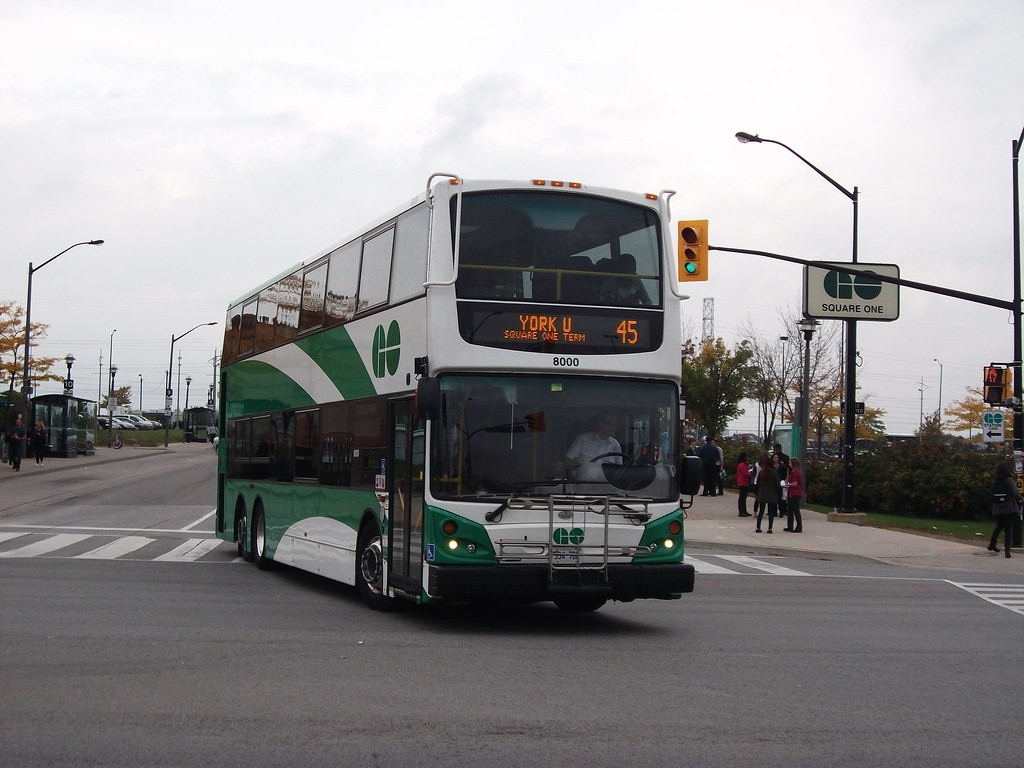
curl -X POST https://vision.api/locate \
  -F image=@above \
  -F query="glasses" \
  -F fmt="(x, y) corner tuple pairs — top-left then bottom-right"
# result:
(605, 420), (619, 427)
(775, 457), (778, 458)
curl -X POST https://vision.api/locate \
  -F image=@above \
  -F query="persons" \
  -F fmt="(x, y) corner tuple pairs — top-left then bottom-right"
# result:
(686, 436), (725, 497)
(600, 254), (653, 307)
(208, 423), (218, 444)
(7, 419), (27, 471)
(986, 464), (1024, 558)
(559, 414), (624, 481)
(737, 444), (803, 533)
(31, 421), (47, 468)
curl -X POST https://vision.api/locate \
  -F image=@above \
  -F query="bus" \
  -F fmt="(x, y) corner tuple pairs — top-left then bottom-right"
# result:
(216, 173), (703, 616)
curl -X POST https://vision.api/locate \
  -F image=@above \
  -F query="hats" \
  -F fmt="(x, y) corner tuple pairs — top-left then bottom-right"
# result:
(720, 469), (727, 481)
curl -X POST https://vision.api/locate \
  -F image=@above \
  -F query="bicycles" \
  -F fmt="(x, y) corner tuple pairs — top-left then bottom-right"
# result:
(112, 428), (123, 449)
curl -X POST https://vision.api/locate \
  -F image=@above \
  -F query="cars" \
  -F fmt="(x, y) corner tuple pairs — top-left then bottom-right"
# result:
(683, 432), (917, 470)
(66, 427), (96, 450)
(97, 414), (164, 430)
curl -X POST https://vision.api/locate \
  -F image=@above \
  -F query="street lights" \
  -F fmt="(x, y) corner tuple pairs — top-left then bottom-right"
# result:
(108, 364), (119, 448)
(109, 329), (117, 397)
(185, 376), (192, 409)
(780, 337), (788, 424)
(795, 318), (823, 510)
(735, 132), (856, 513)
(934, 359), (942, 425)
(64, 353), (76, 390)
(139, 374), (142, 411)
(21, 240), (105, 458)
(165, 322), (217, 447)
(209, 383), (215, 399)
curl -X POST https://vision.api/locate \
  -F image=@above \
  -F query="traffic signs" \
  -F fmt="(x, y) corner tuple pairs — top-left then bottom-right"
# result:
(982, 411), (1004, 442)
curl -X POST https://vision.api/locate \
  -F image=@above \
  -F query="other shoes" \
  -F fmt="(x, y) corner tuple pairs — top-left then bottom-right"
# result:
(756, 529), (762, 533)
(790, 527), (801, 532)
(783, 528), (793, 531)
(1005, 549), (1011, 558)
(988, 544), (1000, 552)
(739, 511), (747, 517)
(779, 511), (788, 518)
(716, 493), (723, 495)
(13, 465), (16, 469)
(752, 512), (757, 518)
(700, 495), (708, 496)
(744, 511), (752, 516)
(35, 464), (39, 467)
(767, 530), (772, 533)
(708, 493), (717, 496)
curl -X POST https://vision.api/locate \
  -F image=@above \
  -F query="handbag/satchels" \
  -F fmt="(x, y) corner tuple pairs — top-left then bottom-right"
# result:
(991, 490), (1010, 503)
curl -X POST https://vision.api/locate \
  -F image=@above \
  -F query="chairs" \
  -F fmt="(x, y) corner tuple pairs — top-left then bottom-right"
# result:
(494, 254), (613, 306)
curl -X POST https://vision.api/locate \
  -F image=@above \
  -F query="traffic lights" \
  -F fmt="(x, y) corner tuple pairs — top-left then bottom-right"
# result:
(678, 220), (709, 281)
(984, 367), (1013, 403)
